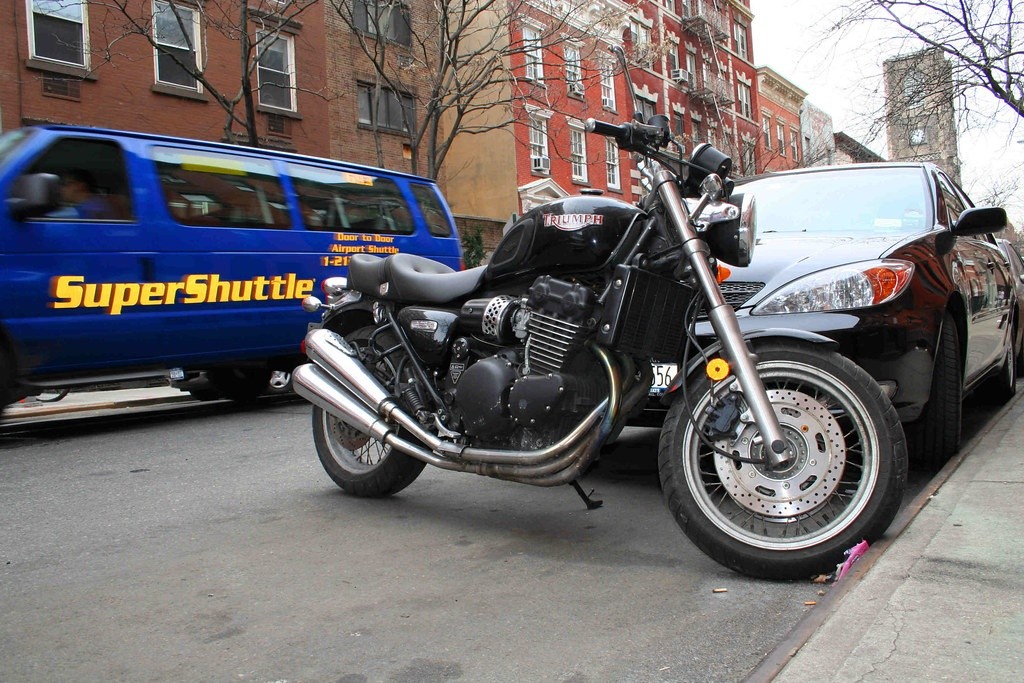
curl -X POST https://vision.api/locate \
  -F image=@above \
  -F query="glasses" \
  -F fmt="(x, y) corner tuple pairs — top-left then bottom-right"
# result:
(60, 180), (74, 186)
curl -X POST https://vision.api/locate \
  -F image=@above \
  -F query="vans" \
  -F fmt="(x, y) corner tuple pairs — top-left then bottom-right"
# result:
(0, 117), (463, 413)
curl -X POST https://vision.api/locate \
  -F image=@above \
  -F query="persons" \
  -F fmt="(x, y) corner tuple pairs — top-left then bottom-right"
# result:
(43, 166), (118, 220)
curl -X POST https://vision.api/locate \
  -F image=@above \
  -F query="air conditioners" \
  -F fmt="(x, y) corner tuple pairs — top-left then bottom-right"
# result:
(569, 81), (585, 95)
(603, 98), (614, 108)
(532, 157), (550, 171)
(671, 68), (689, 83)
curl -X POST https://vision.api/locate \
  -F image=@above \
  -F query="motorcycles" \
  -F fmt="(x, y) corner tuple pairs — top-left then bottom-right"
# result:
(288, 44), (908, 581)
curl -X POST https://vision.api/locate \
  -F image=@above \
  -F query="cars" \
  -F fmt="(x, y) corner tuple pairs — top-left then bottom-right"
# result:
(623, 160), (1024, 467)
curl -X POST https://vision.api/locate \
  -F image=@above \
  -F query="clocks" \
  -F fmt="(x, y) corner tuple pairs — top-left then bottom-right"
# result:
(911, 128), (925, 143)
